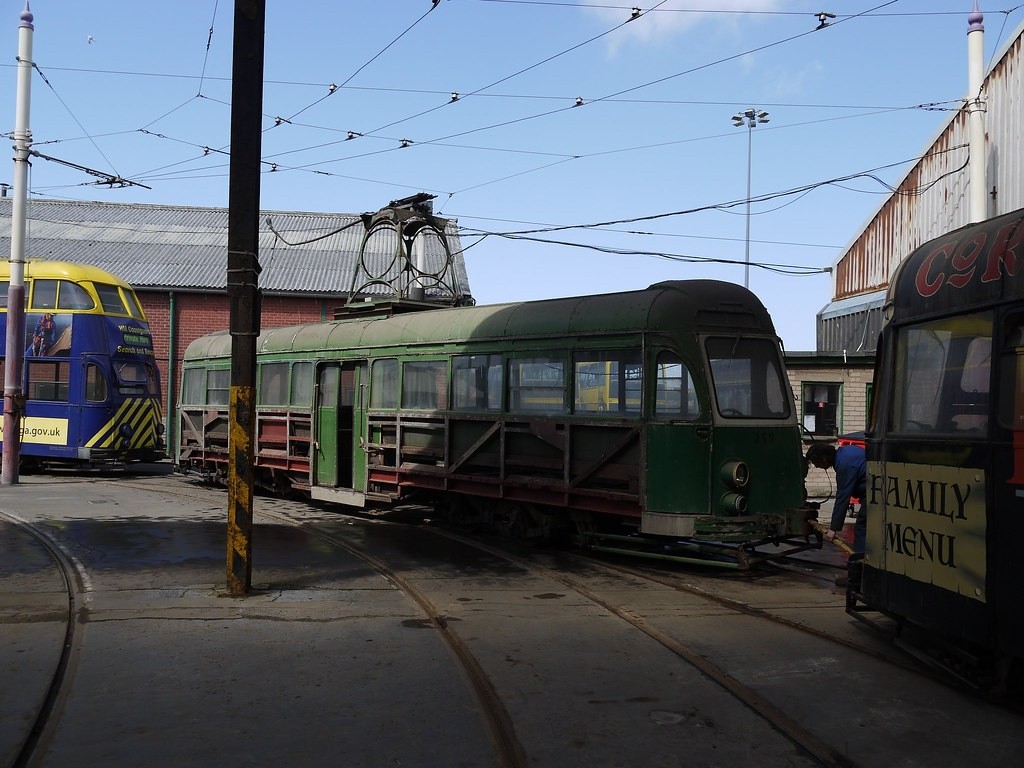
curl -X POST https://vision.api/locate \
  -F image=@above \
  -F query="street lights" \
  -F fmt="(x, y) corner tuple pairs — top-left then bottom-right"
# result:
(729, 108), (771, 292)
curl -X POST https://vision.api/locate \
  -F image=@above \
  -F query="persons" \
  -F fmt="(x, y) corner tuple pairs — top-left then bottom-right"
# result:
(806, 444), (867, 587)
(25, 313), (57, 357)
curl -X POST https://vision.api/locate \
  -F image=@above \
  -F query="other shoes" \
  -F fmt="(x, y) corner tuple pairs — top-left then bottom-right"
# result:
(835, 575), (847, 586)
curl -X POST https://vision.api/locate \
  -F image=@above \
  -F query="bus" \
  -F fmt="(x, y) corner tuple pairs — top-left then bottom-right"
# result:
(0, 258), (163, 472)
(843, 205), (1024, 661)
(167, 193), (825, 569)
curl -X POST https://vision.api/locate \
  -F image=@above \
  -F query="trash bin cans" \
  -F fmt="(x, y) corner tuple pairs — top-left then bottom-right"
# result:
(836, 430), (866, 515)
